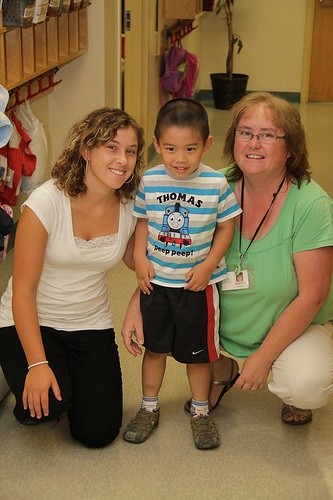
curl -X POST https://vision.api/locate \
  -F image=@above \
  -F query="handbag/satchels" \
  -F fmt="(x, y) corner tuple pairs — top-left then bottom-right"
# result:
(15, 99), (47, 197)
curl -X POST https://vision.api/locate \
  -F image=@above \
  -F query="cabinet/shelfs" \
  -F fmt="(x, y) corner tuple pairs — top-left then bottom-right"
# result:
(0, 6), (89, 92)
(163, 0), (216, 20)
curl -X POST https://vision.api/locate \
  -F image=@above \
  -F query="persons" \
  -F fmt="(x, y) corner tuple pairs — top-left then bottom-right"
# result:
(122, 99), (243, 450)
(0, 107), (145, 448)
(122, 91), (333, 426)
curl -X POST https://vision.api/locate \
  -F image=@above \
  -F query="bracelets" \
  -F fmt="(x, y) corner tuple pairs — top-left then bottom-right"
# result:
(26, 360), (48, 370)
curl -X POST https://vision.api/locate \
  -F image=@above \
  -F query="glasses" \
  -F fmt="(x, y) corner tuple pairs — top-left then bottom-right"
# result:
(234, 130), (285, 144)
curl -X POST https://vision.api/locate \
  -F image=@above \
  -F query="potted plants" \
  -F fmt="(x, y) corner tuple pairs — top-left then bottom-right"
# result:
(209, 0), (250, 112)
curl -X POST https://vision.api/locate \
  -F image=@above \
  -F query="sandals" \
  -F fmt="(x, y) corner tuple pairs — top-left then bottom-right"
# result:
(281, 400), (313, 426)
(183, 358), (241, 414)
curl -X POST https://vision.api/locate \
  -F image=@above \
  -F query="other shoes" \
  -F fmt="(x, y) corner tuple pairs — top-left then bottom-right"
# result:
(190, 408), (220, 449)
(122, 407), (160, 443)
(12, 398), (45, 425)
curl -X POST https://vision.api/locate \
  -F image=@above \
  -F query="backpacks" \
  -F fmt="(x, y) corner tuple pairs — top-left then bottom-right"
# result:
(162, 38), (201, 99)
